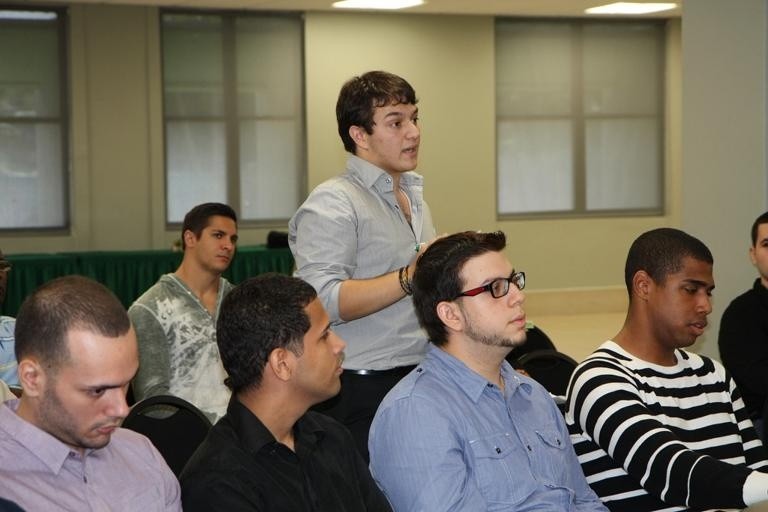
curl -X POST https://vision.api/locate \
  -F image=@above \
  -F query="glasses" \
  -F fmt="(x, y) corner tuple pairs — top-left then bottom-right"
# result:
(453, 271), (526, 303)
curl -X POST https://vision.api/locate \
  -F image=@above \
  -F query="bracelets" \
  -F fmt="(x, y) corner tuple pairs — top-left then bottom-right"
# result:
(399, 265), (413, 295)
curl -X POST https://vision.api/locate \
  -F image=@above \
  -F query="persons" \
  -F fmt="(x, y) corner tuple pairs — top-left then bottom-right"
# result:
(368, 231), (610, 512)
(0, 256), (21, 387)
(127, 205), (238, 427)
(0, 275), (184, 511)
(180, 273), (392, 510)
(718, 213), (767, 449)
(288, 71), (482, 465)
(565, 227), (768, 512)
